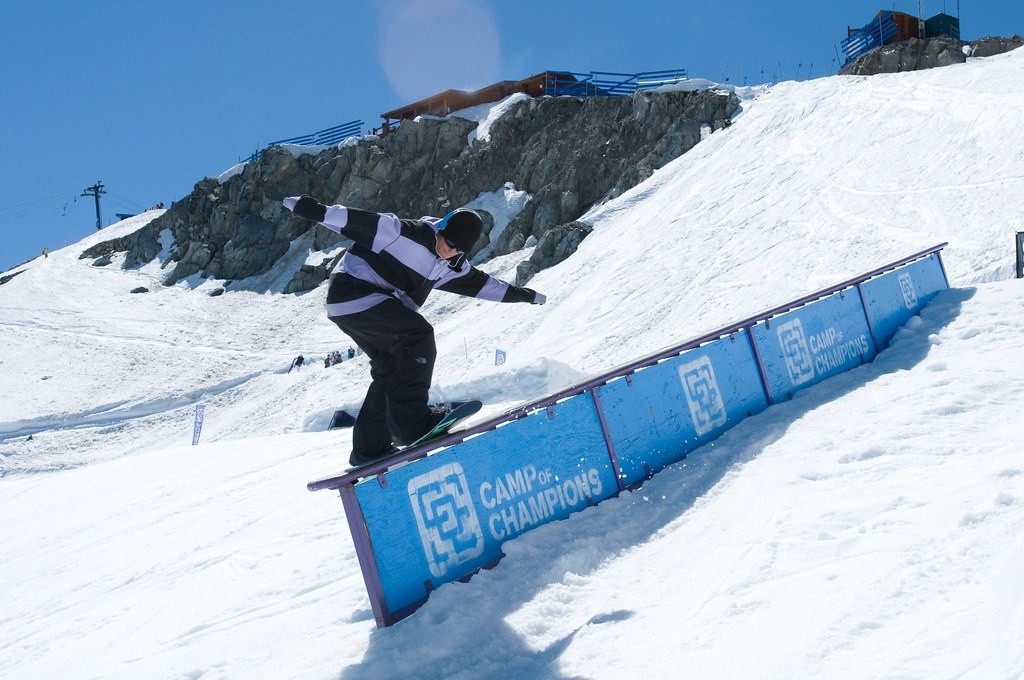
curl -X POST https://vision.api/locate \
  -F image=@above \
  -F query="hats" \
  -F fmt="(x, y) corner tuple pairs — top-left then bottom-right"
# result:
(438, 212), (483, 254)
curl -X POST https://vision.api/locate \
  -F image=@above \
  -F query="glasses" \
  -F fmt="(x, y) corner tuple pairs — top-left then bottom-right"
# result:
(444, 236), (461, 252)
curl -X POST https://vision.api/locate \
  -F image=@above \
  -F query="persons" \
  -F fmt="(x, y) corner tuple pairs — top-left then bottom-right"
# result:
(43, 248), (48, 257)
(280, 194), (547, 469)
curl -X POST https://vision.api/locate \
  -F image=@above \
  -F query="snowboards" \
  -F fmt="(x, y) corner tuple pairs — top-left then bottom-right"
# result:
(346, 395), (486, 471)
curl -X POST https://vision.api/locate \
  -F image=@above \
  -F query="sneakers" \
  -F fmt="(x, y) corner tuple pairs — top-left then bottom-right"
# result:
(399, 412), (448, 444)
(354, 442), (399, 462)
